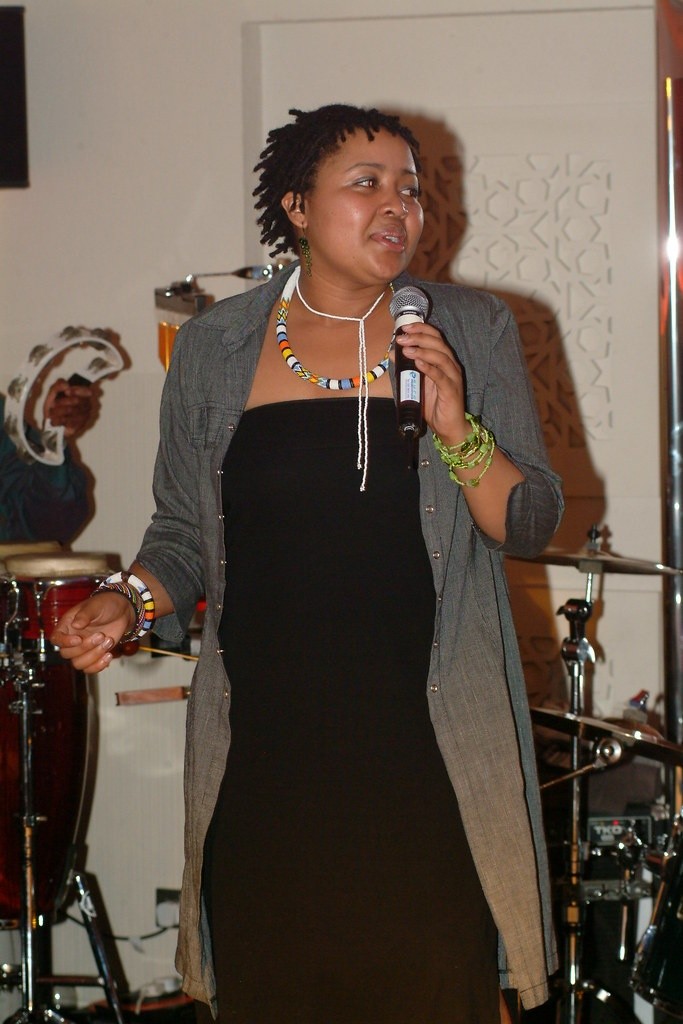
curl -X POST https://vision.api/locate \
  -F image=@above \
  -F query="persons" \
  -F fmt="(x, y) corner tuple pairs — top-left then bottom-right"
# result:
(50, 105), (568, 1024)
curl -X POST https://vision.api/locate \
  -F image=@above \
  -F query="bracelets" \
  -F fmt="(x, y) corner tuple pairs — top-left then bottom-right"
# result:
(433, 412), (495, 487)
(99, 570), (155, 643)
(89, 584), (146, 645)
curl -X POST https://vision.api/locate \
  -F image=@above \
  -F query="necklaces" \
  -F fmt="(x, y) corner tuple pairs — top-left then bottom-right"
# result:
(276, 265), (396, 390)
(296, 266), (385, 491)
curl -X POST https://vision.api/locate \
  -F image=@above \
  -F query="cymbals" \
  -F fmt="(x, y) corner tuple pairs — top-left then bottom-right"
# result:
(528, 705), (683, 767)
(1, 323), (132, 468)
(503, 545), (683, 575)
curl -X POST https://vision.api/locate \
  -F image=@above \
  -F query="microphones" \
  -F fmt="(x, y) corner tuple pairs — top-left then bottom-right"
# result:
(390, 287), (429, 446)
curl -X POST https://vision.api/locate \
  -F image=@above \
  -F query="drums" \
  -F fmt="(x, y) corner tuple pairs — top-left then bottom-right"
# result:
(0, 652), (98, 930)
(5, 551), (109, 642)
